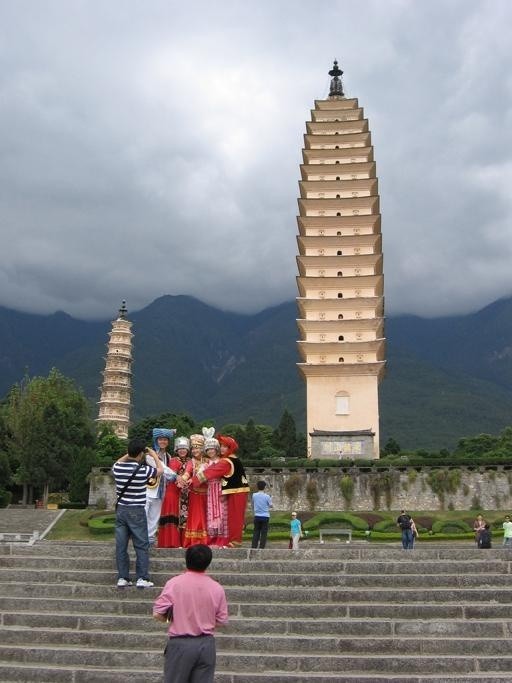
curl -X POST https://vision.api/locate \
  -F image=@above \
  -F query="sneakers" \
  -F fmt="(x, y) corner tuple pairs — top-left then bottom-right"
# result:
(136, 577), (154, 588)
(117, 577), (133, 588)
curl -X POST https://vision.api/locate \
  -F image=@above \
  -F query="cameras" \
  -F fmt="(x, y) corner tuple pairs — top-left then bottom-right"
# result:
(142, 449), (148, 453)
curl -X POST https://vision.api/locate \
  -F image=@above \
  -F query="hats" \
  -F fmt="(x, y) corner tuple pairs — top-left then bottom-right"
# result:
(174, 434), (239, 454)
(292, 512), (296, 516)
(152, 428), (173, 443)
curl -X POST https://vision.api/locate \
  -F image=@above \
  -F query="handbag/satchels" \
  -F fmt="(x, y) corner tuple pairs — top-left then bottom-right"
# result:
(166, 606), (172, 623)
(288, 544), (293, 549)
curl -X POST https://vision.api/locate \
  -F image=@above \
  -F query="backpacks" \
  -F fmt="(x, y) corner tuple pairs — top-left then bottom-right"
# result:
(400, 515), (409, 528)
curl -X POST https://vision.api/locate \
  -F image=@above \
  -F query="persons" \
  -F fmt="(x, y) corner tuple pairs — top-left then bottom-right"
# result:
(289, 511), (303, 550)
(397, 511), (419, 549)
(144, 428), (250, 549)
(473, 515), (486, 548)
(111, 441), (163, 587)
(502, 516), (512, 549)
(478, 525), (492, 549)
(251, 480), (273, 549)
(152, 543), (230, 683)
